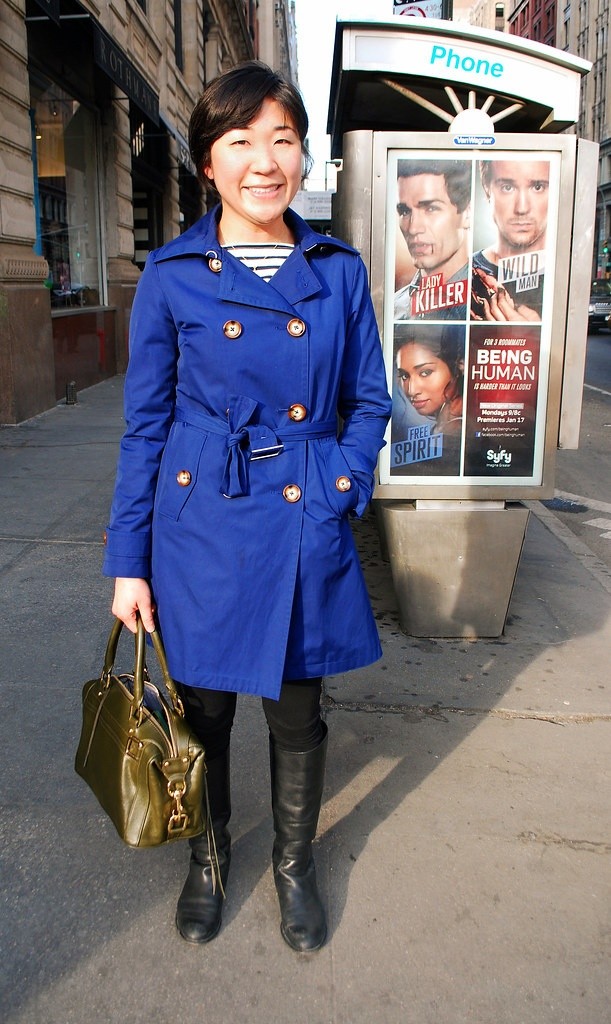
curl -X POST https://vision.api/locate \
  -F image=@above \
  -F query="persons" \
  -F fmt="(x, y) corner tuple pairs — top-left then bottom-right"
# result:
(100, 65), (392, 953)
(393, 324), (466, 435)
(394, 159), (549, 321)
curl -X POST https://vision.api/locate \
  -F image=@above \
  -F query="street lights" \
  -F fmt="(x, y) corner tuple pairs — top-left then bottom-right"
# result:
(325, 161), (342, 191)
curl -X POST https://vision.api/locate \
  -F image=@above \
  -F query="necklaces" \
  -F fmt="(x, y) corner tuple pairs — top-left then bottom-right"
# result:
(218, 223), (287, 272)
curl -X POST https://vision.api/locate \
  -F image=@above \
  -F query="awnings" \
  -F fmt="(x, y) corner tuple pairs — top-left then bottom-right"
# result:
(25, 0), (198, 177)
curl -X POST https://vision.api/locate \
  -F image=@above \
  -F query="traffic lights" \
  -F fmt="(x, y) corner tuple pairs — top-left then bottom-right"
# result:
(603, 243), (607, 255)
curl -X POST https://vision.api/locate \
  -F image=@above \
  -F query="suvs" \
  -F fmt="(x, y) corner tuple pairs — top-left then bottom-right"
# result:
(588, 278), (611, 335)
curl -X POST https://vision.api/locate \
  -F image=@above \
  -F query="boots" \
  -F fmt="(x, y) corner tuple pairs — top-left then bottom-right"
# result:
(175, 750), (232, 943)
(269, 719), (329, 950)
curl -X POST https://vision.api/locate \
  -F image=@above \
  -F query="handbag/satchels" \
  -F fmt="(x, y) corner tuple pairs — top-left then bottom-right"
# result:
(75, 616), (206, 848)
